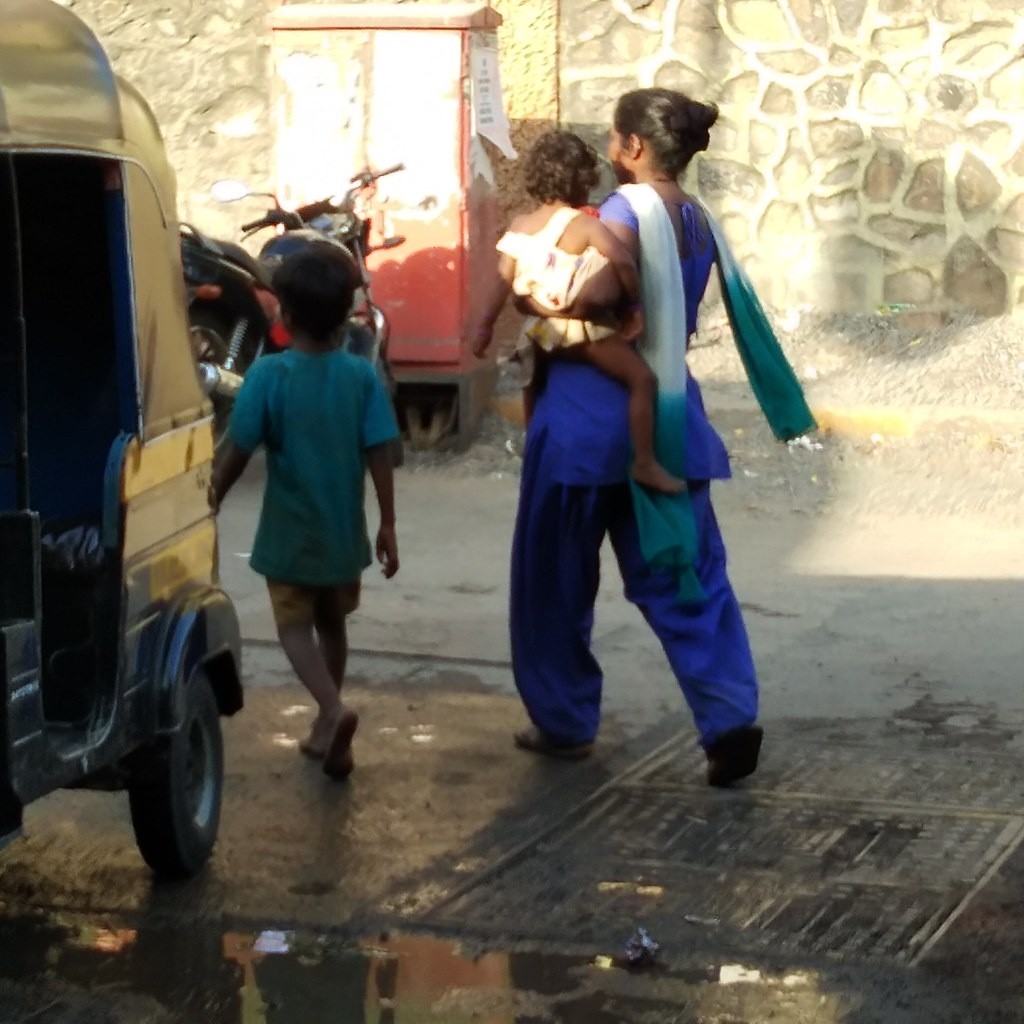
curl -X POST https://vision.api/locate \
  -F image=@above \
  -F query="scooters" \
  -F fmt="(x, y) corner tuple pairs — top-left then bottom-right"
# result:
(180, 165), (403, 446)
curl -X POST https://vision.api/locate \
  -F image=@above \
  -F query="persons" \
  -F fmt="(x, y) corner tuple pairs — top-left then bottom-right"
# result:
(508, 88), (820, 788)
(471, 130), (685, 493)
(212, 229), (400, 778)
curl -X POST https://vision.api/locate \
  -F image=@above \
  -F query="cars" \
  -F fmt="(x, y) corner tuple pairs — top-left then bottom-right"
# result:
(0, 0), (244, 876)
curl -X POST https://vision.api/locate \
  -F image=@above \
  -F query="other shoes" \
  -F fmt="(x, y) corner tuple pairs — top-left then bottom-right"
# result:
(514, 726), (594, 760)
(707, 725), (762, 786)
